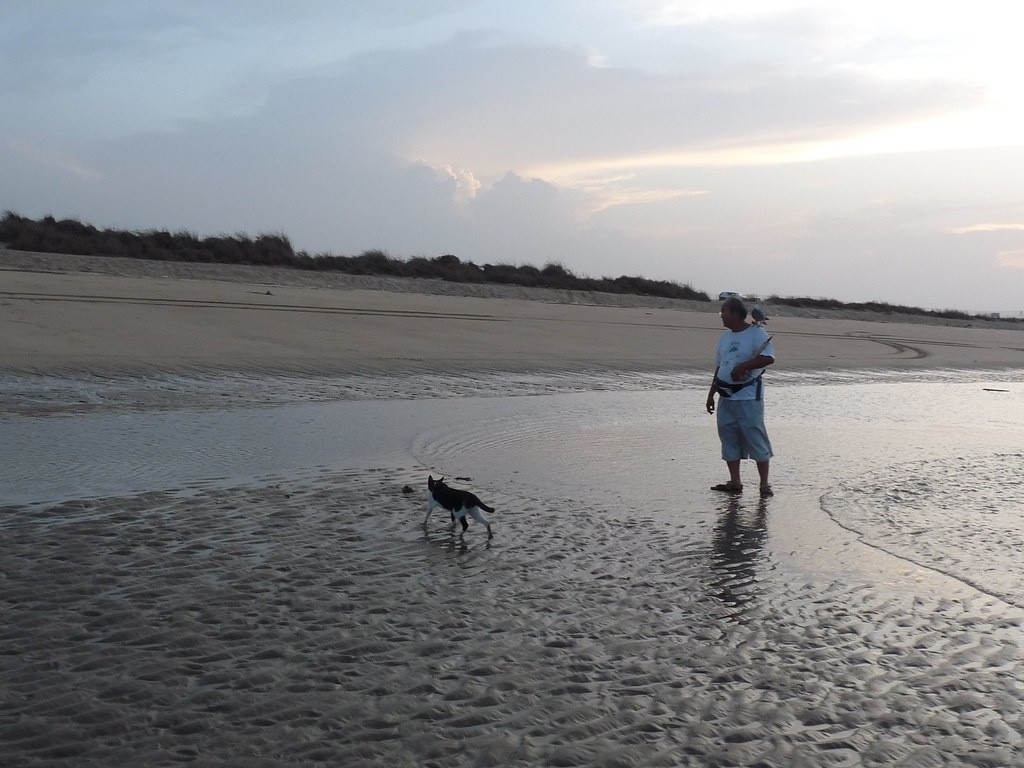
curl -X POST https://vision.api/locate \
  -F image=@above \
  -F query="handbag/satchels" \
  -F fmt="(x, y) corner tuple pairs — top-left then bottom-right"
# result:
(713, 369), (766, 400)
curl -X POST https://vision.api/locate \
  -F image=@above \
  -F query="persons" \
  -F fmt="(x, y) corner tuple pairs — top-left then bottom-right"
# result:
(706, 297), (775, 497)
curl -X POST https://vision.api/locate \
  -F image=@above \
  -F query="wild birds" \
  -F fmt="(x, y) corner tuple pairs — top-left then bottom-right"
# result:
(750, 305), (769, 326)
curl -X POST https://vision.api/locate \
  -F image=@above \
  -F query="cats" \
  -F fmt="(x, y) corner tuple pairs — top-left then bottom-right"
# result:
(423, 474), (495, 539)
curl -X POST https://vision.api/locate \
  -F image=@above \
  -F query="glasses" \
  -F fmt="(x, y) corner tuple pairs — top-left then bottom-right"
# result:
(718, 310), (734, 316)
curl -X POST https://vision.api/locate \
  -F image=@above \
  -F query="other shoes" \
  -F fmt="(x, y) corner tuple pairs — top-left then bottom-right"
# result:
(716, 481), (743, 491)
(760, 484), (773, 498)
